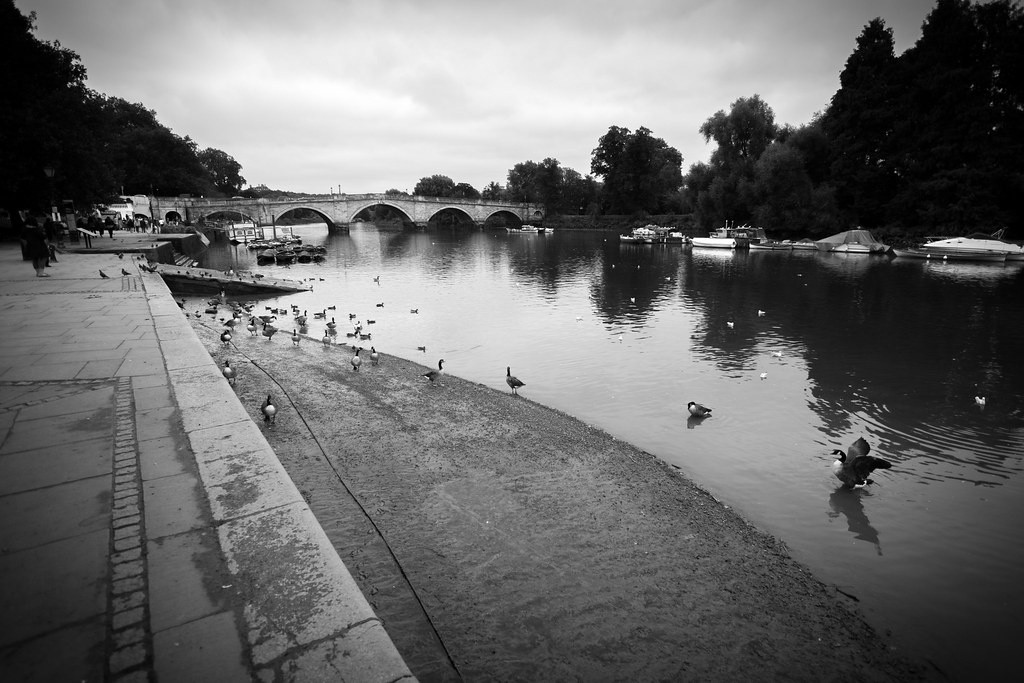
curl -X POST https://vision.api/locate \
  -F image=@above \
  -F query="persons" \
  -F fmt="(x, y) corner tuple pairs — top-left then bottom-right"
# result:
(15, 211), (52, 277)
(159, 217), (164, 226)
(198, 215), (203, 223)
(122, 216), (158, 233)
(43, 217), (66, 249)
(76, 215), (117, 239)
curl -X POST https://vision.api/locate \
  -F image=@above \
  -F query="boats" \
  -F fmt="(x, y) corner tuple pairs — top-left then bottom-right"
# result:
(619, 223), (692, 245)
(505, 224), (555, 233)
(692, 219), (1024, 264)
(228, 213), (328, 265)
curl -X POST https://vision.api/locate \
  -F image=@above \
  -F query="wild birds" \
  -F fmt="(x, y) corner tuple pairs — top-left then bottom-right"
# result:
(508, 238), (990, 406)
(261, 394), (278, 424)
(351, 347), (363, 372)
(221, 330), (232, 346)
(424, 358), (447, 387)
(98, 269), (111, 279)
(829, 437), (892, 491)
(112, 236), (419, 338)
(223, 360), (235, 385)
(370, 346), (379, 366)
(292, 329), (301, 346)
(506, 366), (526, 395)
(687, 401), (714, 419)
(418, 345), (425, 352)
(321, 330), (332, 348)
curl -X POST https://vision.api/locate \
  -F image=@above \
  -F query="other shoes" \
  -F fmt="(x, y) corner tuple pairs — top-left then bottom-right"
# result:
(36, 272), (52, 278)
